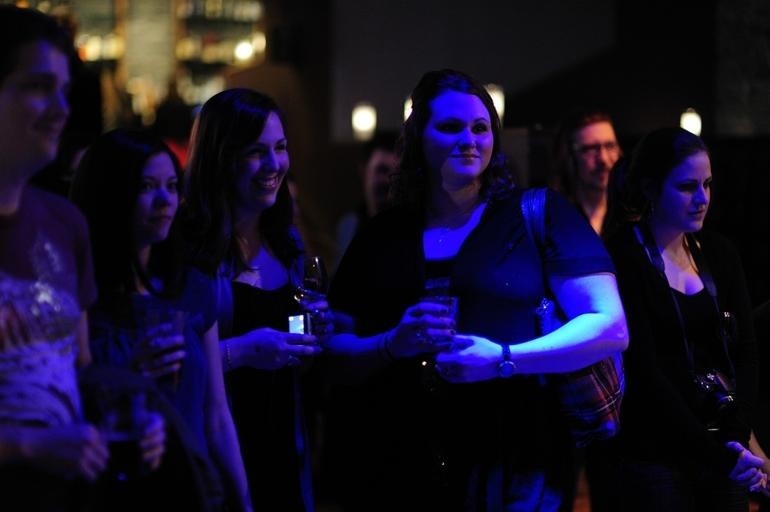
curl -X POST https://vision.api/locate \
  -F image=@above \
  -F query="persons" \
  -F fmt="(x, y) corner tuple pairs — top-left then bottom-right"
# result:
(1, 0), (770, 510)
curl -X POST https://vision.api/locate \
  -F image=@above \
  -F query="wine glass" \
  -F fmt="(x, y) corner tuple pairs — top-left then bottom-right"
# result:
(289, 256), (329, 339)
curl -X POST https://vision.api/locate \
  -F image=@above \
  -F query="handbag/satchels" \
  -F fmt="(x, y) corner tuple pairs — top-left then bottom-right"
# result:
(534, 298), (626, 437)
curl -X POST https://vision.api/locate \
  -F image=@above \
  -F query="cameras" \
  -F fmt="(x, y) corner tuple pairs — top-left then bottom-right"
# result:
(689, 365), (739, 422)
(287, 311), (312, 346)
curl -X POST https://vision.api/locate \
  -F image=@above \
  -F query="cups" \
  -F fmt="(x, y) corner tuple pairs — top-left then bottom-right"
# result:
(145, 322), (186, 391)
(419, 295), (459, 347)
